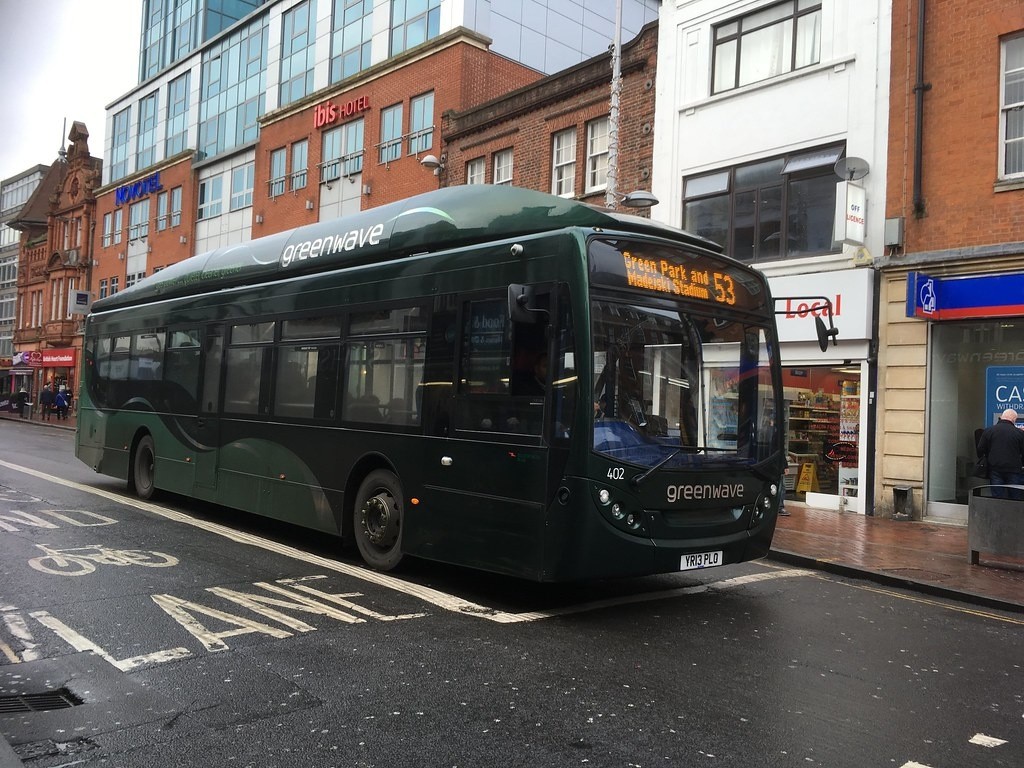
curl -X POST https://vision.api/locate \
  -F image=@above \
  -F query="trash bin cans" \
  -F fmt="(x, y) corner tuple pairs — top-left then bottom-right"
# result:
(23, 403), (34, 420)
(968, 484), (1024, 564)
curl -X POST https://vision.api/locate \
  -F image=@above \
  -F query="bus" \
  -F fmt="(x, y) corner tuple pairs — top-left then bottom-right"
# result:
(74, 185), (840, 586)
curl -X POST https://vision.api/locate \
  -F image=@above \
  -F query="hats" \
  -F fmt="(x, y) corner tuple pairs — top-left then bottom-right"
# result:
(20, 388), (27, 392)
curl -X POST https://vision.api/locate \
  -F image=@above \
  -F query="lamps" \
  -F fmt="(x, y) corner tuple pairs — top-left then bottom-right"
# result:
(420, 154), (445, 176)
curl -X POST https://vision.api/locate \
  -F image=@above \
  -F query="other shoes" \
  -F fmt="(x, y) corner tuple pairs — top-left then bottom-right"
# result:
(42, 418), (45, 420)
(65, 415), (68, 418)
(47, 418), (50, 420)
(57, 417), (60, 419)
(63, 417), (66, 420)
(778, 508), (791, 516)
(38, 402), (42, 413)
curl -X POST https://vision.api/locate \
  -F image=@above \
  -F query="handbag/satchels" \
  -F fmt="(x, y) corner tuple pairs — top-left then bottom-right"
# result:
(64, 400), (69, 407)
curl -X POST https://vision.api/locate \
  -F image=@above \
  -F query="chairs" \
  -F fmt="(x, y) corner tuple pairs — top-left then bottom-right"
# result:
(97, 347), (407, 423)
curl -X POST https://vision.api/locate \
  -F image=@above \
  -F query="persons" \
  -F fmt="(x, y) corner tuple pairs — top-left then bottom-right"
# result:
(40, 385), (73, 421)
(518, 344), (601, 436)
(766, 413), (792, 516)
(978, 409), (1024, 500)
